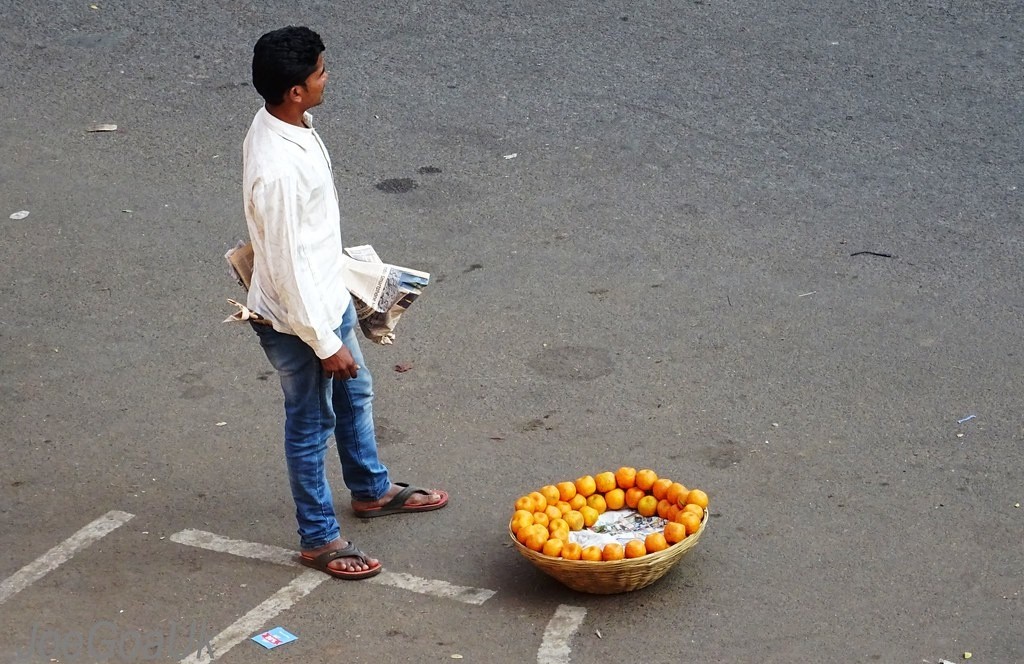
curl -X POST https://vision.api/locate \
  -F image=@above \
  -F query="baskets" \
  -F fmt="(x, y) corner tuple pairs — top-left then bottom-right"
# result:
(508, 502), (708, 593)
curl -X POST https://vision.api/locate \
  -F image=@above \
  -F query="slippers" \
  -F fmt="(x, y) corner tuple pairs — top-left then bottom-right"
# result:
(354, 482), (448, 518)
(298, 537), (381, 578)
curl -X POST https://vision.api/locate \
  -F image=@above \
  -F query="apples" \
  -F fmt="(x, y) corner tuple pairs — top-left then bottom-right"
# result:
(511, 466), (708, 562)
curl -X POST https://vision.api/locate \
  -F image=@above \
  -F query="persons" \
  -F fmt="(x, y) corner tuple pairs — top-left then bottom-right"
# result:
(242, 26), (449, 581)
